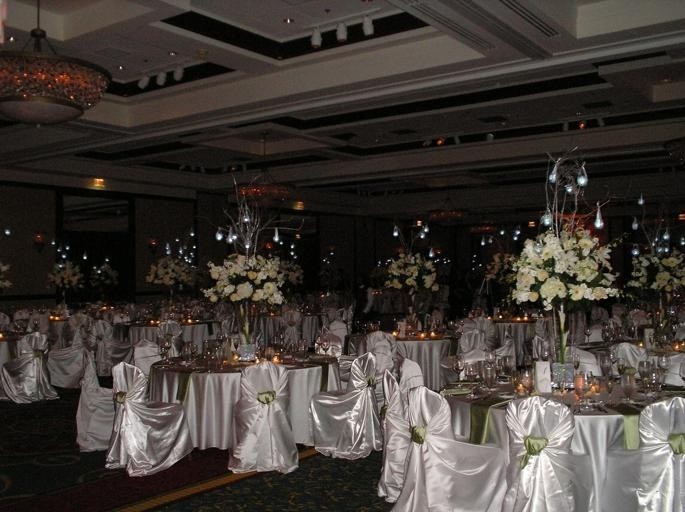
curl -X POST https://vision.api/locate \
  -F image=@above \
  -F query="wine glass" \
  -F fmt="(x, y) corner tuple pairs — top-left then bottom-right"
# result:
(157, 327), (330, 376)
(453, 321), (675, 413)
(354, 316), (456, 339)
(13, 319), (39, 336)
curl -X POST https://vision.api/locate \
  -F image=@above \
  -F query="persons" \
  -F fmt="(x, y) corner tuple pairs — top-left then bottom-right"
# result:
(352, 272), (382, 334)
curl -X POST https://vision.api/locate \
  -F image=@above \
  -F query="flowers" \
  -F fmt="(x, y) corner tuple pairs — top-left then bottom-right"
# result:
(483, 225), (683, 311)
(46, 257), (84, 292)
(146, 253), (197, 295)
(0, 264), (15, 296)
(200, 252), (305, 307)
(383, 253), (446, 294)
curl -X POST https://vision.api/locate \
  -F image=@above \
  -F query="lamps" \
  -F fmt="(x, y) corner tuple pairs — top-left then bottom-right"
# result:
(179, 158), (253, 176)
(425, 174), (469, 224)
(233, 122), (290, 207)
(1, 0), (114, 124)
(421, 126), (509, 145)
(469, 209), (504, 235)
(556, 112), (615, 130)
(135, 58), (194, 92)
(302, 6), (386, 46)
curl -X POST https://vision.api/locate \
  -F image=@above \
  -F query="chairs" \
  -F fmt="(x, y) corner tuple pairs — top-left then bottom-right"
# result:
(0, 296), (683, 511)
